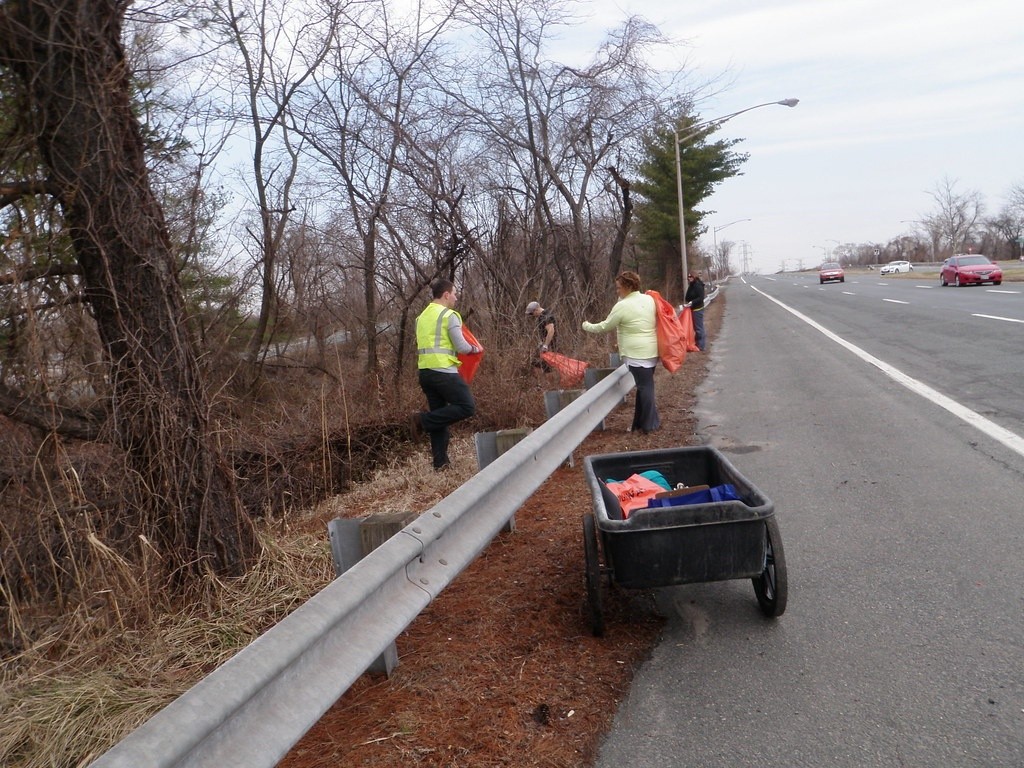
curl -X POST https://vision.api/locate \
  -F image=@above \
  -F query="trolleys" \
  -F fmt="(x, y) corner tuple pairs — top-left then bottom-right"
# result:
(580, 442), (790, 637)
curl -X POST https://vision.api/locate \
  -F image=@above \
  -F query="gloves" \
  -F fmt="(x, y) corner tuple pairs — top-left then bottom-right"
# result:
(472, 345), (479, 353)
(540, 345), (548, 352)
(679, 305), (683, 311)
(683, 301), (692, 307)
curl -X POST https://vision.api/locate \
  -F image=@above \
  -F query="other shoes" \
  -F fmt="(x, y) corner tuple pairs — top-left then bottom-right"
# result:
(645, 426), (662, 434)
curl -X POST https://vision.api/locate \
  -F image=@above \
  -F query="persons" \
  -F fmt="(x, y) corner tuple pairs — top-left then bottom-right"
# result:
(526, 302), (557, 387)
(684, 271), (706, 350)
(582, 271), (661, 433)
(409, 281), (480, 471)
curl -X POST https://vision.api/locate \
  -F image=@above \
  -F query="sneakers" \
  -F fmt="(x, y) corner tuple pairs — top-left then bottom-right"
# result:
(436, 462), (459, 471)
(410, 413), (420, 444)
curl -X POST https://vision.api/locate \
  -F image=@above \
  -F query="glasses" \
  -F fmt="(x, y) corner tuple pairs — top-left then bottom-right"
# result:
(529, 309), (535, 315)
(688, 275), (692, 278)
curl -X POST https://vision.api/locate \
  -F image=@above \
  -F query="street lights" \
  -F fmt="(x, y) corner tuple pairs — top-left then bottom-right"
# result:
(713, 217), (752, 285)
(898, 220), (935, 266)
(673, 96), (801, 307)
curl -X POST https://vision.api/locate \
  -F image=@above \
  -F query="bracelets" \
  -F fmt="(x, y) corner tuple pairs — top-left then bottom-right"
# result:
(471, 345), (477, 354)
(543, 345), (548, 349)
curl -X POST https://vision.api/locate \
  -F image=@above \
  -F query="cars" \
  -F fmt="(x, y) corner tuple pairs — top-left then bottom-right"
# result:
(938, 252), (1002, 288)
(818, 262), (847, 284)
(880, 260), (914, 276)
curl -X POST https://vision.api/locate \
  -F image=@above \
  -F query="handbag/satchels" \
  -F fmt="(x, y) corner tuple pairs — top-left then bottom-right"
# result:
(648, 484), (741, 507)
(605, 474), (667, 520)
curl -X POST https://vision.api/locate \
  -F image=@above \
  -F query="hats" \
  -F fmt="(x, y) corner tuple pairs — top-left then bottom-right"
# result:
(525, 302), (540, 315)
